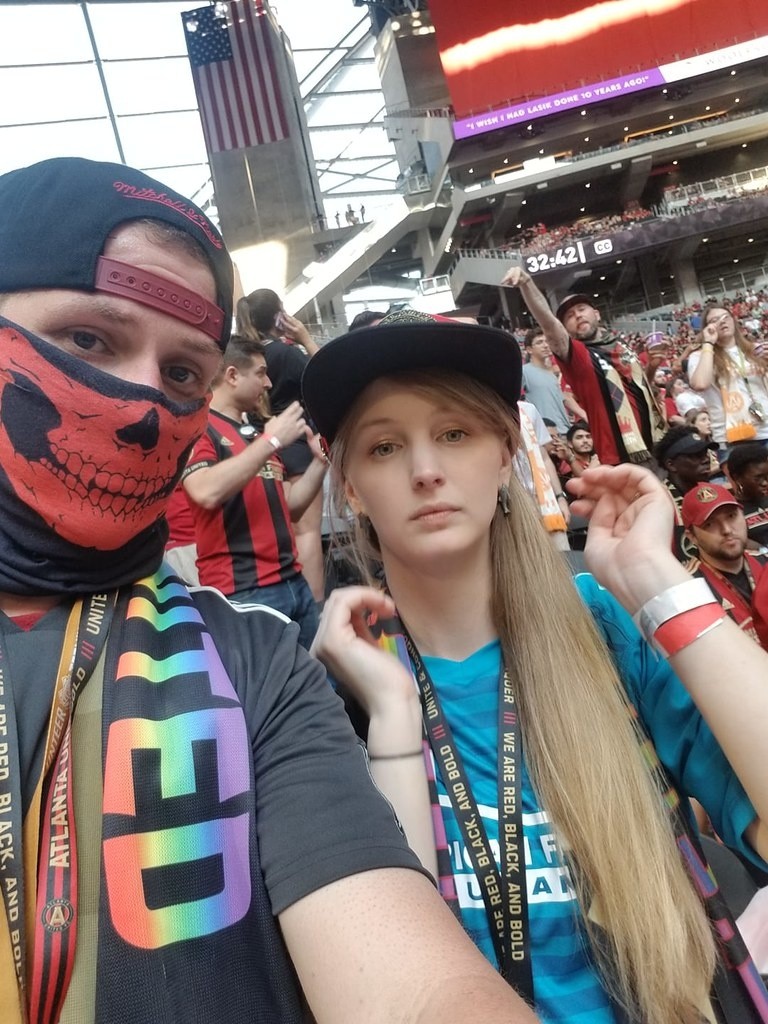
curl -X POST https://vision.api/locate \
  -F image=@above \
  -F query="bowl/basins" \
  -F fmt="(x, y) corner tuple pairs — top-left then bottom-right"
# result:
(645, 332), (665, 351)
(754, 344), (764, 358)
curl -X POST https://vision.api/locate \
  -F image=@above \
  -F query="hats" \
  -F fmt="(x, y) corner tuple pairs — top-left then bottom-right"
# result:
(1, 156), (234, 353)
(556, 293), (598, 319)
(652, 425), (720, 467)
(300, 310), (523, 461)
(680, 481), (744, 531)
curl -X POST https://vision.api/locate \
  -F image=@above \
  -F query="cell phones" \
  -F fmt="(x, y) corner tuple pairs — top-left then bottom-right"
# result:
(275, 312), (286, 332)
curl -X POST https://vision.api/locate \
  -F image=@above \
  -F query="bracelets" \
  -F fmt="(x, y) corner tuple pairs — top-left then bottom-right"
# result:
(260, 433), (283, 452)
(633, 577), (726, 660)
(364, 749), (425, 760)
(701, 348), (715, 355)
(701, 340), (714, 346)
(567, 453), (576, 464)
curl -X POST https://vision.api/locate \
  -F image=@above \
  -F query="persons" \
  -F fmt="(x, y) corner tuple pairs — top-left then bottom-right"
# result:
(0, 160), (560, 1023)
(174, 164), (768, 648)
(296, 307), (768, 1024)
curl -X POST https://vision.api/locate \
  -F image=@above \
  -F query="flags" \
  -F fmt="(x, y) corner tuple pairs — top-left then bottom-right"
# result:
(180, 0), (289, 154)
